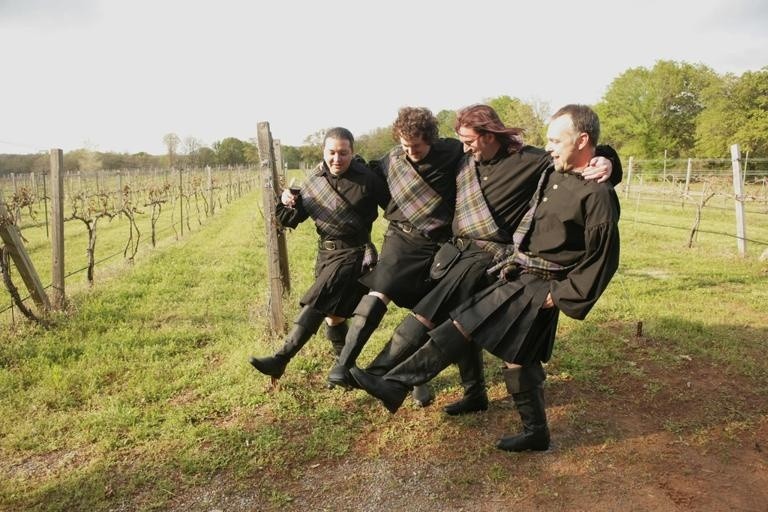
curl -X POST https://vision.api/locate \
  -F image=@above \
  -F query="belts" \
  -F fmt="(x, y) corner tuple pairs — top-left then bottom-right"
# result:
(453, 236), (482, 251)
(392, 222), (425, 235)
(317, 233), (372, 251)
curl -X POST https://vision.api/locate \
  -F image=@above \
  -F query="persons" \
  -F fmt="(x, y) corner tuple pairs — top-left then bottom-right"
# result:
(316, 106), (528, 414)
(248, 128), (388, 383)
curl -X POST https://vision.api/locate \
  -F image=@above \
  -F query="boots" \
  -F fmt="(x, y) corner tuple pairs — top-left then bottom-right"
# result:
(248, 304), (323, 379)
(444, 348), (487, 415)
(325, 293), (388, 386)
(348, 314), (470, 415)
(497, 361), (551, 452)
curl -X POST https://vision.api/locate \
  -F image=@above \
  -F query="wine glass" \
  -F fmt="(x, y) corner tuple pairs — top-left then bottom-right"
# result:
(282, 178), (303, 210)
